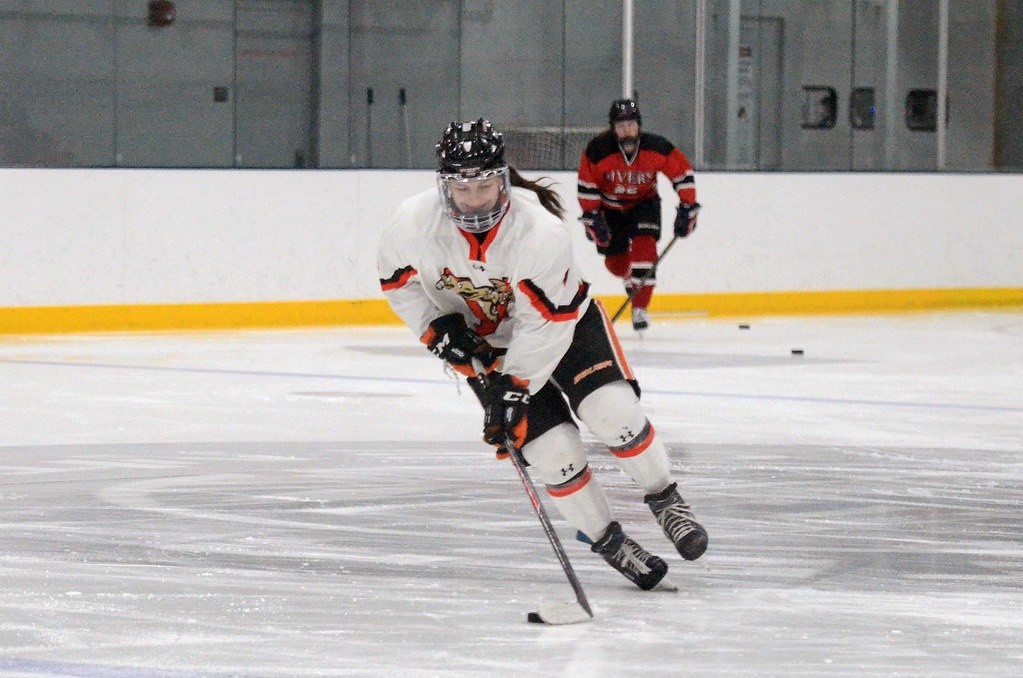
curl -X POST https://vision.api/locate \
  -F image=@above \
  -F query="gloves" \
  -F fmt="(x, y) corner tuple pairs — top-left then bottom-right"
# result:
(578, 211), (612, 254)
(420, 313), (508, 379)
(482, 374), (531, 460)
(674, 203), (701, 237)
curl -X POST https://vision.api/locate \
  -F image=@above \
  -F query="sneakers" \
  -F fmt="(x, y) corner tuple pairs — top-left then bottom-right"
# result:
(644, 482), (715, 571)
(576, 521), (680, 591)
(624, 278), (633, 297)
(631, 306), (649, 341)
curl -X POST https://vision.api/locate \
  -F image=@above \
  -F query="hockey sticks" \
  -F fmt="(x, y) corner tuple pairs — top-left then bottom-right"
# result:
(610, 236), (679, 323)
(471, 357), (594, 626)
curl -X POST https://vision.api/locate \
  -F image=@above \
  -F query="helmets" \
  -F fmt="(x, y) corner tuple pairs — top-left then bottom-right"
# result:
(434, 118), (511, 233)
(609, 100), (642, 133)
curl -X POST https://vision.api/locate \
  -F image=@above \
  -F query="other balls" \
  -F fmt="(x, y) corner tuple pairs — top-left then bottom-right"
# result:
(791, 349), (803, 355)
(739, 325), (750, 330)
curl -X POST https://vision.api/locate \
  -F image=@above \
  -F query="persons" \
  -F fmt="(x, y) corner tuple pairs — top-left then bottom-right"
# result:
(375, 119), (708, 594)
(577, 99), (700, 328)
